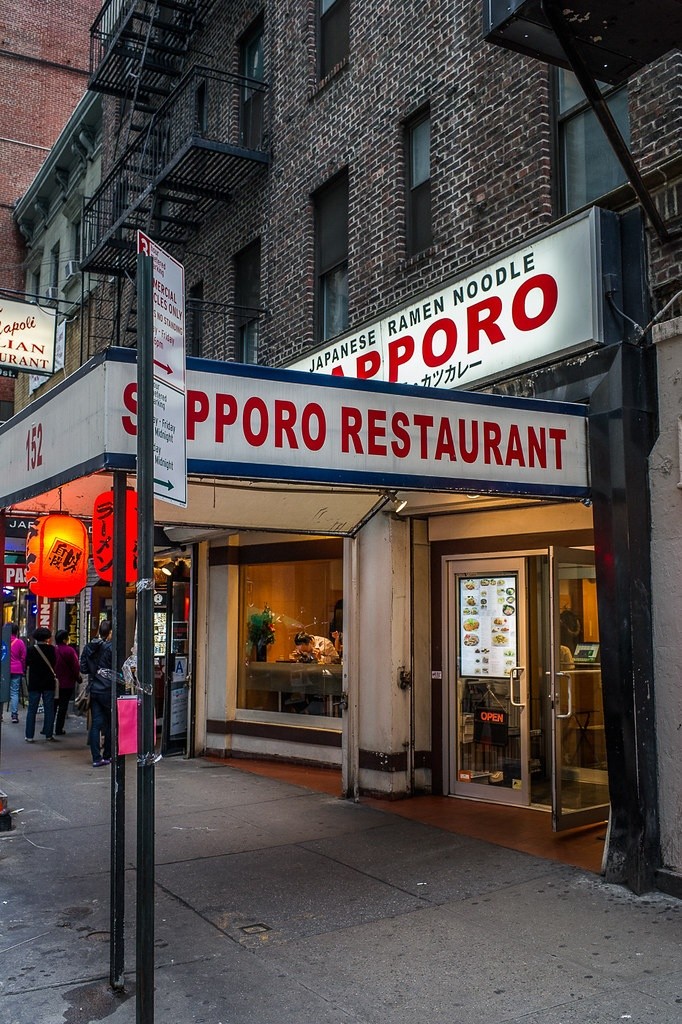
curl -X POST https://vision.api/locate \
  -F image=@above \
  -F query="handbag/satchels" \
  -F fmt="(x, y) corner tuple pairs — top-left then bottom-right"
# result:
(73, 687), (90, 716)
(75, 674), (82, 684)
(55, 678), (59, 699)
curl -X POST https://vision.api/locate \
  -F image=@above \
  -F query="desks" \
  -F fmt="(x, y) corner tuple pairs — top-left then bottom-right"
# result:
(246, 661), (344, 718)
(568, 708), (603, 770)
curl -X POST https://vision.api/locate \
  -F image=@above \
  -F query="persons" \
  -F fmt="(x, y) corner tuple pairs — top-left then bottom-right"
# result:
(332, 631), (343, 653)
(81, 619), (112, 766)
(283, 632), (340, 713)
(1, 622), (27, 722)
(560, 645), (575, 670)
(40, 628), (81, 736)
(24, 626), (58, 743)
(560, 610), (581, 643)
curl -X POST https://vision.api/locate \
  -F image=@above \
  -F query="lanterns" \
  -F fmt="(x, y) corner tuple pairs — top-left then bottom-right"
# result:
(26, 510), (90, 600)
(92, 486), (137, 584)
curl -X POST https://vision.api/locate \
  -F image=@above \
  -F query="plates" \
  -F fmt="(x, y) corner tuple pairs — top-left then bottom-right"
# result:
(491, 642), (509, 647)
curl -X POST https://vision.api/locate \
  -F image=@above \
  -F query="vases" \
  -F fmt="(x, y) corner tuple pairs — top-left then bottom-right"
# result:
(256, 645), (267, 662)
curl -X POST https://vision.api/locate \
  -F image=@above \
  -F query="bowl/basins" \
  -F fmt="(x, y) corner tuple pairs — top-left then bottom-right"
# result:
(463, 634), (479, 646)
(506, 588), (515, 595)
(463, 618), (479, 632)
(481, 579), (489, 586)
(503, 605), (514, 616)
(506, 596), (514, 603)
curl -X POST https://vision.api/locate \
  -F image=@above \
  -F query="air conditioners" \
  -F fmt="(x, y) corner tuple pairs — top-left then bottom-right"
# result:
(45, 287), (58, 304)
(64, 261), (80, 279)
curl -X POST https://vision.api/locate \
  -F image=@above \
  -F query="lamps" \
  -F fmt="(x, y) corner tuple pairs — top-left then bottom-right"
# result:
(161, 557), (178, 576)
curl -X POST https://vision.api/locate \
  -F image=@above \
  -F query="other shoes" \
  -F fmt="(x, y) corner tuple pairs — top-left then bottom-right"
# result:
(109, 758), (112, 763)
(56, 730), (65, 735)
(25, 737), (33, 741)
(40, 731), (44, 734)
(11, 714), (19, 722)
(45, 737), (55, 742)
(92, 759), (110, 767)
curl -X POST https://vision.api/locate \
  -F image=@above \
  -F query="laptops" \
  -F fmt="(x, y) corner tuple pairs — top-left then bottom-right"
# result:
(572, 642), (600, 663)
